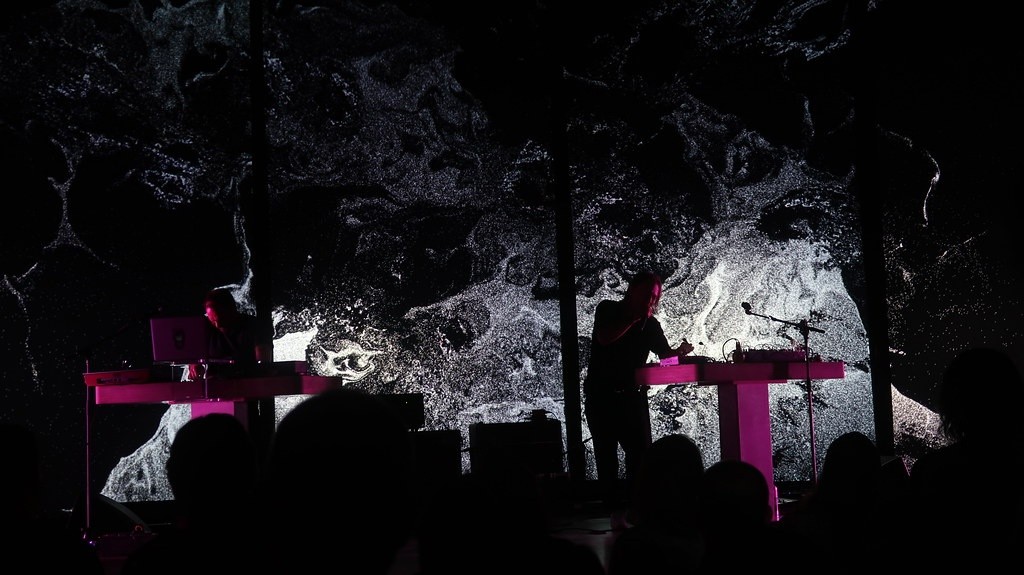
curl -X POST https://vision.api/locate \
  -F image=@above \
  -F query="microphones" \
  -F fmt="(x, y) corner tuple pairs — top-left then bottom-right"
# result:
(640, 316), (648, 331)
(120, 307), (163, 334)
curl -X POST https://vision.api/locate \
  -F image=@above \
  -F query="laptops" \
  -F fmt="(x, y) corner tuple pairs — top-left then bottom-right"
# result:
(151, 318), (237, 364)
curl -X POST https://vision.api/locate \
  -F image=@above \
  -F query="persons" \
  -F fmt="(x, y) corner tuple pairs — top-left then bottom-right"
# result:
(0, 348), (1023, 575)
(584, 271), (692, 529)
(202, 289), (274, 444)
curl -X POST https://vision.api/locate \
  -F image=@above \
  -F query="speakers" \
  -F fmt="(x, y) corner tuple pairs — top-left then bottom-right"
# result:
(879, 455), (910, 480)
(70, 494), (152, 536)
(410, 430), (462, 506)
(469, 419), (569, 504)
